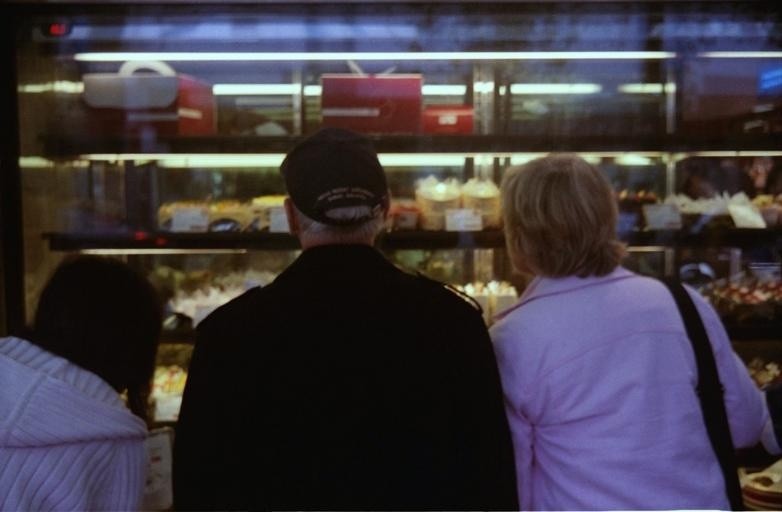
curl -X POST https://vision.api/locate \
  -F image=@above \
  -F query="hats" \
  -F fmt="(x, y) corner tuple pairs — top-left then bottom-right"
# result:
(279, 127), (388, 227)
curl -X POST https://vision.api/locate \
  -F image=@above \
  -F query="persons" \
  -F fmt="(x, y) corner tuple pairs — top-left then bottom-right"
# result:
(481, 151), (766, 509)
(167, 124), (520, 509)
(0, 252), (162, 509)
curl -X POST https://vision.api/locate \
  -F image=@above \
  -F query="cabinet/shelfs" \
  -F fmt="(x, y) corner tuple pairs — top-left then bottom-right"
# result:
(37, 1), (782, 432)
